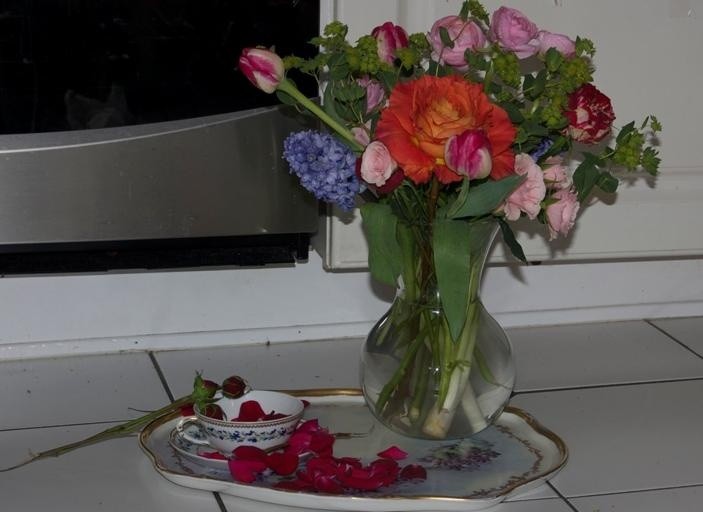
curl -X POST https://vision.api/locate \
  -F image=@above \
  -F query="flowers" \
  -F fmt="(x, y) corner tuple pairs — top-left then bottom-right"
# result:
(239, 0), (663, 439)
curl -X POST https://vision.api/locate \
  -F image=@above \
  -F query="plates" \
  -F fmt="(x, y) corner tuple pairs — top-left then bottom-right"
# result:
(168, 425), (316, 472)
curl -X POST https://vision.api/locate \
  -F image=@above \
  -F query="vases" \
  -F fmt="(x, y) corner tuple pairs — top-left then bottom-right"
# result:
(359, 212), (516, 439)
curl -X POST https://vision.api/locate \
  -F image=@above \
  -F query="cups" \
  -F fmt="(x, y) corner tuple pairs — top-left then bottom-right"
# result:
(176, 387), (303, 454)
